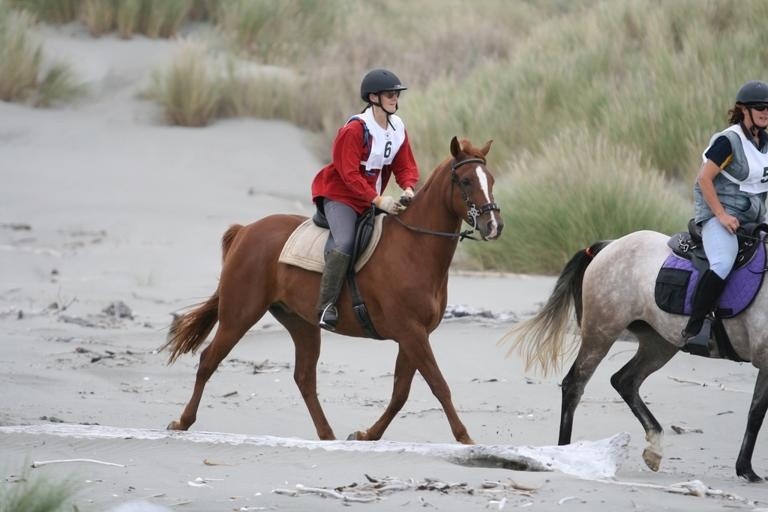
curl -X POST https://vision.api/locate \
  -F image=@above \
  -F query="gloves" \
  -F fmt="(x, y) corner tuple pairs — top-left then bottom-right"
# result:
(375, 189), (415, 217)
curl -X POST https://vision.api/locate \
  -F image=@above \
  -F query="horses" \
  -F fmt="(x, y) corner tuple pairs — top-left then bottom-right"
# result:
(495, 230), (768, 482)
(157, 137), (504, 445)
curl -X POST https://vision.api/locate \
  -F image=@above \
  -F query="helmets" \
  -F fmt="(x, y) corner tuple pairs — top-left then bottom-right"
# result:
(361, 69), (408, 103)
(735, 80), (768, 104)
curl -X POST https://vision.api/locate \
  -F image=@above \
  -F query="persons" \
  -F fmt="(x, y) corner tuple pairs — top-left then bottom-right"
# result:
(682, 81), (768, 354)
(310, 67), (420, 329)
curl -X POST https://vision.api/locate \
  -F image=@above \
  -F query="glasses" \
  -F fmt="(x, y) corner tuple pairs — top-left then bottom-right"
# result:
(746, 102), (768, 111)
(382, 90), (400, 99)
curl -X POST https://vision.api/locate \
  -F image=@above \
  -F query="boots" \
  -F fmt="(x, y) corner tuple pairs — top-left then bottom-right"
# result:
(681, 269), (726, 339)
(317, 246), (352, 325)
